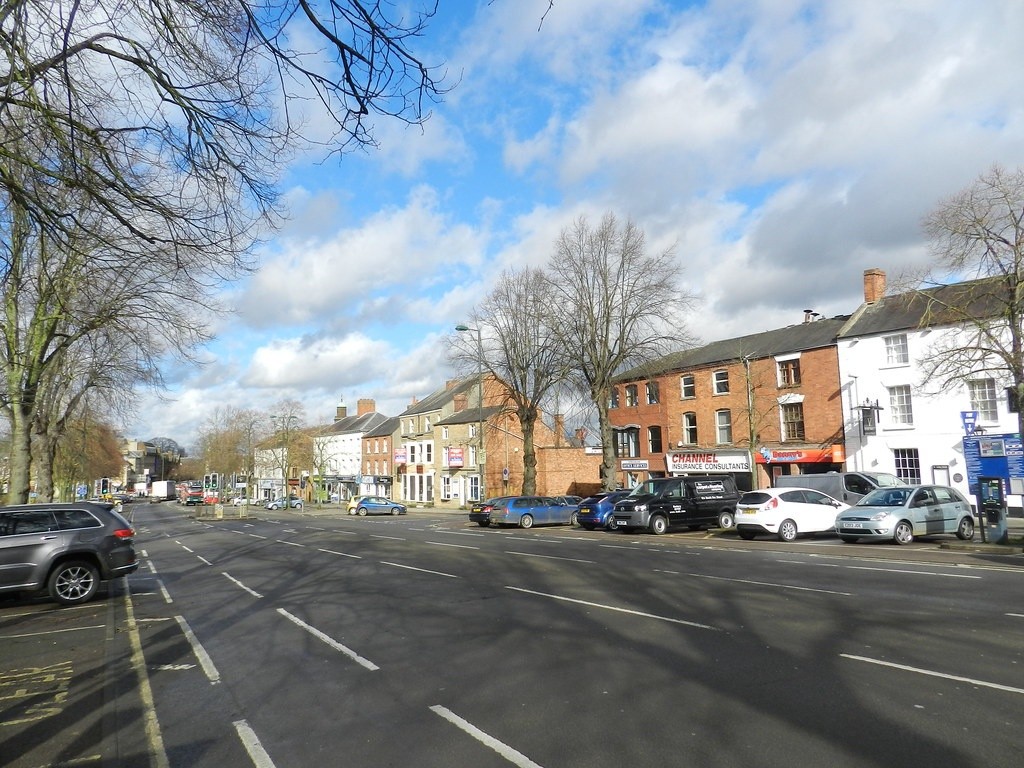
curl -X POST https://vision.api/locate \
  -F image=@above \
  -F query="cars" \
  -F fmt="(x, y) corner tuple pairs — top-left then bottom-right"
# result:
(265, 496), (303, 510)
(91, 494), (130, 505)
(733, 486), (853, 542)
(346, 495), (407, 516)
(127, 489), (136, 497)
(834, 483), (975, 544)
(223, 493), (241, 501)
(467, 490), (648, 530)
(230, 495), (263, 506)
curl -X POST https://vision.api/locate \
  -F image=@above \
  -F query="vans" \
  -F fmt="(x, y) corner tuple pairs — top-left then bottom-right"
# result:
(774, 474), (912, 506)
(613, 476), (740, 535)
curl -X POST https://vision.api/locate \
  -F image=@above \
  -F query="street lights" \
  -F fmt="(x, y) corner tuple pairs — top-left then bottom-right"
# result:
(455, 325), (485, 502)
(270, 415), (298, 509)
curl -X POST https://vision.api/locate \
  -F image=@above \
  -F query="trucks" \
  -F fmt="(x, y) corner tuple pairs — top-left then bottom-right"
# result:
(177, 481), (205, 506)
(134, 482), (151, 497)
(151, 480), (177, 502)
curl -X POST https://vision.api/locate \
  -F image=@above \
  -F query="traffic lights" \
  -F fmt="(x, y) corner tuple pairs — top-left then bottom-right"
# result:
(211, 474), (218, 489)
(204, 475), (210, 489)
(102, 478), (109, 494)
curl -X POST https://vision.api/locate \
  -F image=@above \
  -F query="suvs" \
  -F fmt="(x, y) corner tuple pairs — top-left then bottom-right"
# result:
(0, 502), (140, 607)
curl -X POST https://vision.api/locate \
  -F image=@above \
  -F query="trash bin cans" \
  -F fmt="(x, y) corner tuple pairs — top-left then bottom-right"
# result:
(983, 501), (1008, 545)
(116, 502), (123, 513)
(466, 500), (480, 510)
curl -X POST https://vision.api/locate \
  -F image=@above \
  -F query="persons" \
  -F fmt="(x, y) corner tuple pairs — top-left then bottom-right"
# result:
(631, 477), (637, 489)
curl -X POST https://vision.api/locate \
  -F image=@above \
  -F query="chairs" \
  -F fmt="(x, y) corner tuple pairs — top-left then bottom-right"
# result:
(914, 492), (929, 507)
(884, 491), (902, 505)
